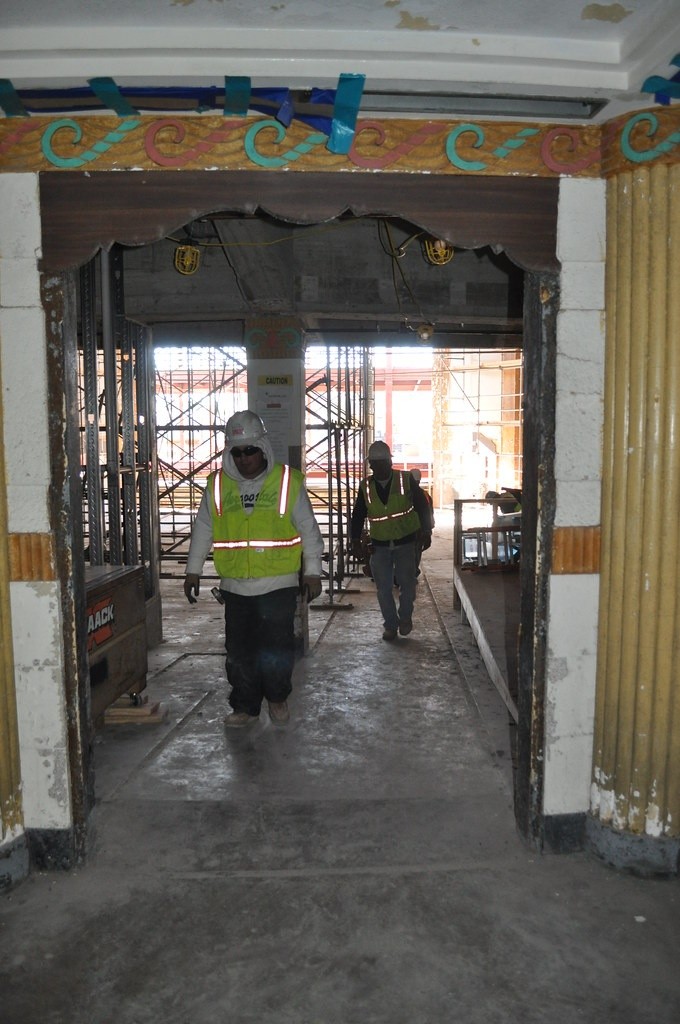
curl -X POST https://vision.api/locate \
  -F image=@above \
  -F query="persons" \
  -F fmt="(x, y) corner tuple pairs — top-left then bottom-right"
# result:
(350, 440), (435, 640)
(485, 492), (521, 560)
(183, 411), (325, 728)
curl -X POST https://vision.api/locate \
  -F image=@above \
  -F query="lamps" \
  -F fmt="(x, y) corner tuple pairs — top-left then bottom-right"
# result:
(175, 245), (200, 275)
(415, 325), (433, 344)
(425, 236), (454, 264)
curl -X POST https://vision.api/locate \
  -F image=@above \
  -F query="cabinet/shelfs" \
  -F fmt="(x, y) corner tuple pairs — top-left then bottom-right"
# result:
(83, 564), (146, 724)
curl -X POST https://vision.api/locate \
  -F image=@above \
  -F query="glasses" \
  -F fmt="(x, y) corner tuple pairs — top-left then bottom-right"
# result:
(230, 446), (260, 457)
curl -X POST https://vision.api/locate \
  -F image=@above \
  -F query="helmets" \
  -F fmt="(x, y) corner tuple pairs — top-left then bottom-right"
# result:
(225, 410), (268, 442)
(486, 491), (498, 498)
(410, 469), (421, 480)
(366, 441), (393, 460)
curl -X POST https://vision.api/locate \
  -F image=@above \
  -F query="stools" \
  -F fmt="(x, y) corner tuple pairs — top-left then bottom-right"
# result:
(468, 526), (519, 567)
(463, 531), (491, 559)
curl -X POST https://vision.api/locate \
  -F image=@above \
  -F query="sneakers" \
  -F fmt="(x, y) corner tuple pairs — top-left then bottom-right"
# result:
(224, 710), (260, 728)
(383, 628), (397, 640)
(268, 700), (290, 726)
(400, 621), (412, 635)
